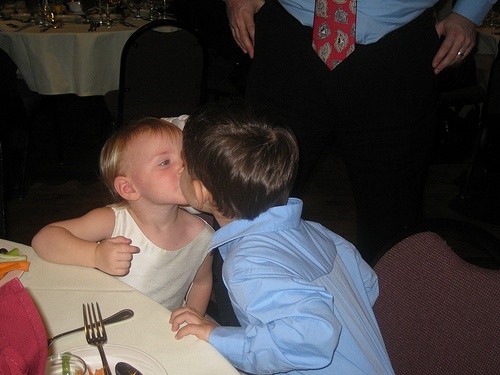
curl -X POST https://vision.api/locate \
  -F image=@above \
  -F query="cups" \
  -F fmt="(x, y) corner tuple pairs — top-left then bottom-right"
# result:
(2, 4), (26, 16)
(47, 354), (90, 375)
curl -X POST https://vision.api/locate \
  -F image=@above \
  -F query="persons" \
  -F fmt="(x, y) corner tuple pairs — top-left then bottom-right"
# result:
(169, 107), (394, 375)
(226, 0), (496, 269)
(31, 117), (217, 317)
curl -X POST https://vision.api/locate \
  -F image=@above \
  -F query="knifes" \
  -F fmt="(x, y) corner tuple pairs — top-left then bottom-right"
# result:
(115, 362), (143, 375)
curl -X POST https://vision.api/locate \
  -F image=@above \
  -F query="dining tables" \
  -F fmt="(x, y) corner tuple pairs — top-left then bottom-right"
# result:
(0, 11), (182, 97)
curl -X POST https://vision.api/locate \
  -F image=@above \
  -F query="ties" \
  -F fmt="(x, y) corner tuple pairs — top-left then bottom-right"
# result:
(312, 0), (357, 72)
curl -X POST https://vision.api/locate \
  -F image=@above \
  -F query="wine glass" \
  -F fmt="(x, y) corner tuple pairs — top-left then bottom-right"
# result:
(27, 0), (170, 28)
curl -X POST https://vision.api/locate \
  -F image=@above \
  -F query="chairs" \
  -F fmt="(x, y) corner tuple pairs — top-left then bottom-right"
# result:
(371, 32), (500, 375)
(0, 19), (210, 201)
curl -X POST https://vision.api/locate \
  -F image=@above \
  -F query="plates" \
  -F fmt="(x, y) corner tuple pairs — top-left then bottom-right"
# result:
(59, 344), (169, 375)
(0, 242), (25, 287)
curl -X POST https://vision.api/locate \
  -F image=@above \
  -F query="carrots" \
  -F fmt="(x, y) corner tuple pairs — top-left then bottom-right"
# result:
(0, 260), (30, 278)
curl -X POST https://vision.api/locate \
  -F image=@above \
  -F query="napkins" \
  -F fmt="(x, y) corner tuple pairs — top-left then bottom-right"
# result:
(0, 277), (48, 375)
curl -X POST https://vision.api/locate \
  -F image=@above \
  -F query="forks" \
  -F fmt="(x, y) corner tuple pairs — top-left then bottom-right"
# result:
(82, 301), (112, 375)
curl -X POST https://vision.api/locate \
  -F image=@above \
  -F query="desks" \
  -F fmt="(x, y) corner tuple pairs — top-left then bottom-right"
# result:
(0, 239), (243, 375)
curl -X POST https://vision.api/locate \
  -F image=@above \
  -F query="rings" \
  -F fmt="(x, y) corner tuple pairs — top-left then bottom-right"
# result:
(457, 51), (464, 57)
